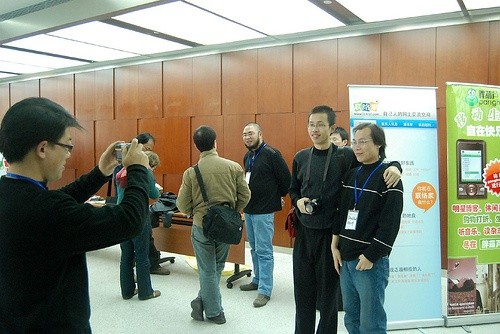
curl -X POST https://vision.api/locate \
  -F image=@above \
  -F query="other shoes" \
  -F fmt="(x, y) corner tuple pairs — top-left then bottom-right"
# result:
(148, 290), (161, 299)
(134, 288), (138, 295)
(253, 294), (270, 307)
(191, 297), (205, 321)
(240, 282), (258, 291)
(151, 266), (170, 275)
(206, 312), (226, 324)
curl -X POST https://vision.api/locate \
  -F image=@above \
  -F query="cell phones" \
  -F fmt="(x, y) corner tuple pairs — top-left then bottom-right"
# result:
(456, 139), (487, 199)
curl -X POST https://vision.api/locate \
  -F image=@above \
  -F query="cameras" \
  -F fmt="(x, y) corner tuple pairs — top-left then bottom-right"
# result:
(115, 143), (131, 161)
(305, 197), (320, 215)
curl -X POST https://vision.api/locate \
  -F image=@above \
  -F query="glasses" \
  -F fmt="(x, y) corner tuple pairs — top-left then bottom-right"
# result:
(144, 145), (152, 151)
(37, 136), (73, 153)
(351, 140), (373, 146)
(307, 123), (331, 128)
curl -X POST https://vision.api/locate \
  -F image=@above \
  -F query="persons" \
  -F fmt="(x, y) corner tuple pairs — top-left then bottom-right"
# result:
(331, 122), (404, 334)
(239, 123), (292, 307)
(289, 105), (403, 334)
(176, 126), (251, 324)
(113, 132), (169, 301)
(0, 97), (149, 334)
(330, 127), (348, 146)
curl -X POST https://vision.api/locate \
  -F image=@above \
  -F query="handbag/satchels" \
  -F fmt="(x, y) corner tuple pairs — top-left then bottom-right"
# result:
(203, 201), (243, 245)
(285, 208), (298, 238)
